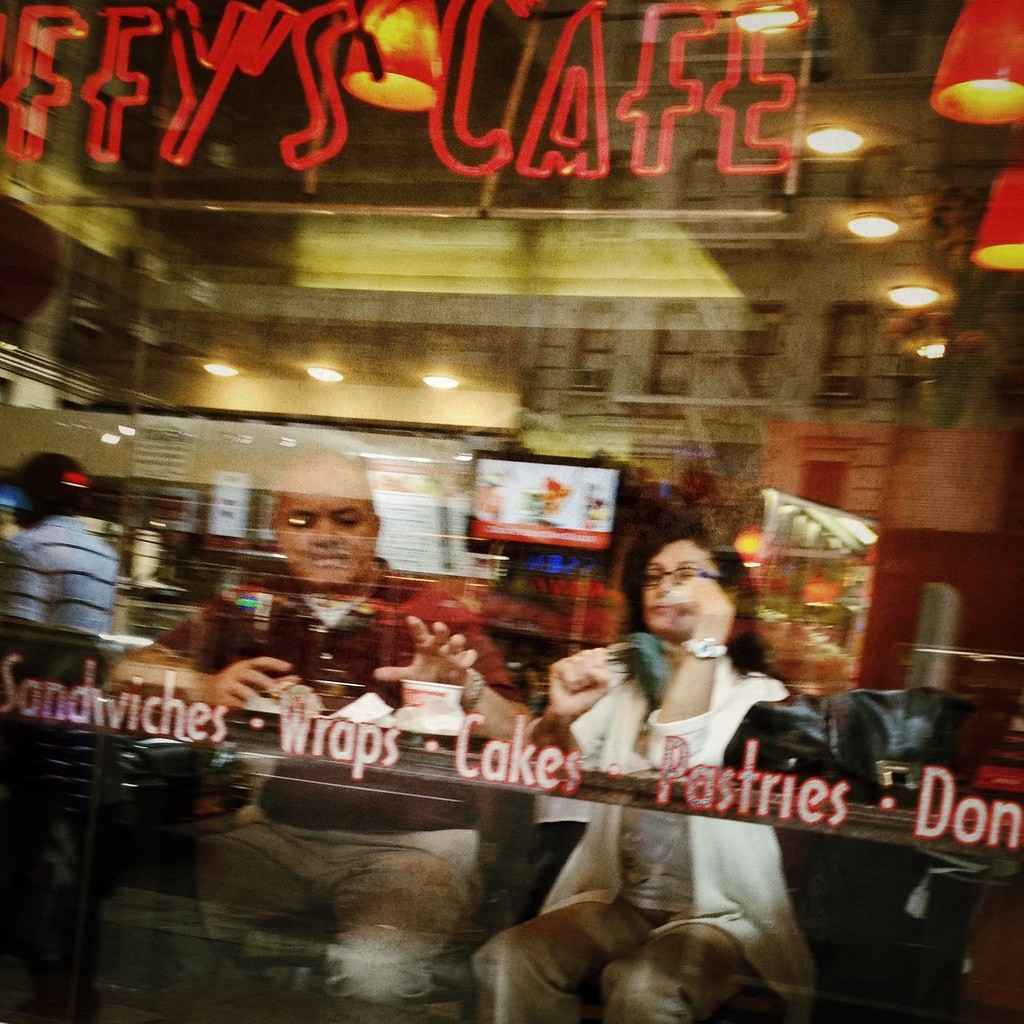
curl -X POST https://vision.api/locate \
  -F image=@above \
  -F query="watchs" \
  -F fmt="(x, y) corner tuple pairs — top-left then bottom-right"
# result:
(679, 638), (728, 659)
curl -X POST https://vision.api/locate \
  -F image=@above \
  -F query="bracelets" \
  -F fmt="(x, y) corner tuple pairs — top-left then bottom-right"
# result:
(466, 668), (488, 716)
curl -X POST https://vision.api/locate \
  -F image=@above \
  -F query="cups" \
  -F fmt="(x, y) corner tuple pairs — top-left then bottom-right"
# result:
(401, 680), (465, 708)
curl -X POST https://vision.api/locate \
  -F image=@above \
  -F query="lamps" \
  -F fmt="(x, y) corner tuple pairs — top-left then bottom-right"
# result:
(734, 528), (770, 569)
(969, 167), (1024, 271)
(927, 0), (1024, 126)
(802, 578), (840, 607)
(341, 0), (446, 112)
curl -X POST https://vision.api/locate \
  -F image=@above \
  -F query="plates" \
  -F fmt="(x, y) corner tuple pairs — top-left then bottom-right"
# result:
(244, 708), (345, 727)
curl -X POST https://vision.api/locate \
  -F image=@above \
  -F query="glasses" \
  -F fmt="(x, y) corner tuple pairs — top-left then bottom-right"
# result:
(642, 567), (725, 586)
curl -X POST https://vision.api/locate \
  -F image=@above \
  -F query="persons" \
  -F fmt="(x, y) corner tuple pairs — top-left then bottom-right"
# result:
(470, 522), (817, 1024)
(96, 445), (536, 1024)
(0, 450), (119, 1024)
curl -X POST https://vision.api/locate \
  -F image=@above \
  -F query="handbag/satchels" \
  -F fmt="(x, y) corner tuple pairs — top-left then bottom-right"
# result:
(721, 679), (977, 804)
